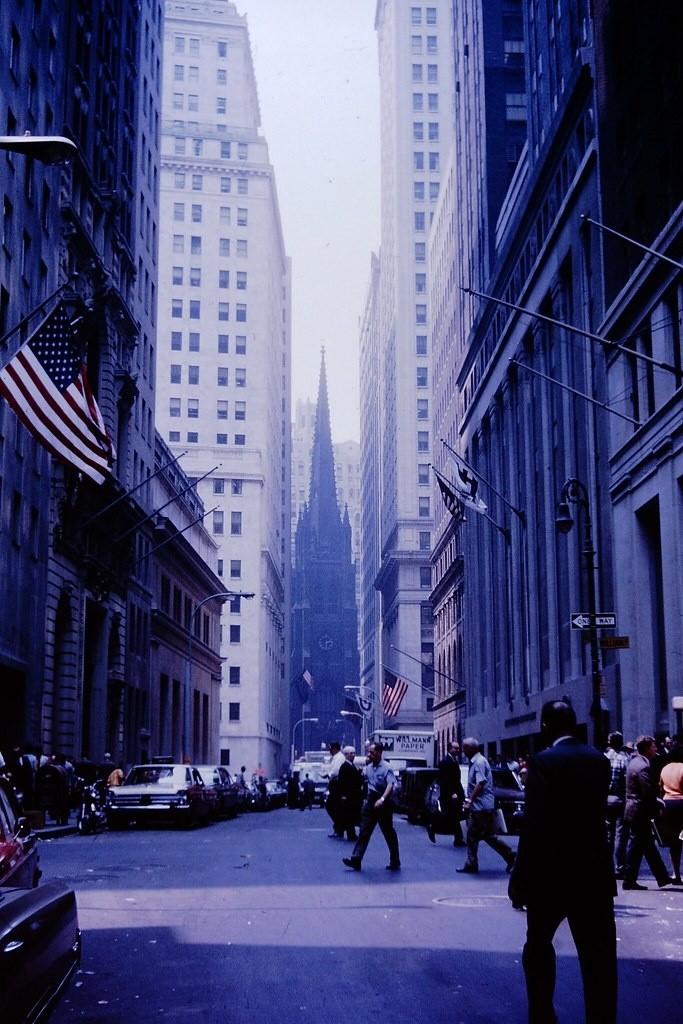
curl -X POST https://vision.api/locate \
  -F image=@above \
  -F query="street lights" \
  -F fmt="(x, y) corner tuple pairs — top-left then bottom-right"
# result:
(344, 685), (380, 729)
(182, 592), (255, 763)
(554, 477), (605, 751)
(293, 718), (318, 764)
(335, 711), (365, 756)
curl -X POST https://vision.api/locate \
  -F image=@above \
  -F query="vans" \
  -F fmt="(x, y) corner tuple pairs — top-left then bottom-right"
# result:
(402, 767), (437, 824)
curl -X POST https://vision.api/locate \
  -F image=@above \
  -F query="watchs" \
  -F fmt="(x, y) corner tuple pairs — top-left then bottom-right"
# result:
(380, 797), (385, 801)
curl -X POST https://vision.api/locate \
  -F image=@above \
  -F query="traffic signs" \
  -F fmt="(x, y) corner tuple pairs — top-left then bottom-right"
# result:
(570, 613), (617, 630)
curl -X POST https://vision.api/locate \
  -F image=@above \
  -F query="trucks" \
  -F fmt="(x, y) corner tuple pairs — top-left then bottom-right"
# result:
(295, 751), (371, 808)
(369, 729), (434, 811)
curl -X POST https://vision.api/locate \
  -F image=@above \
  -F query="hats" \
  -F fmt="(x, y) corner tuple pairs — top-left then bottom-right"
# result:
(104, 752), (111, 757)
(623, 741), (635, 750)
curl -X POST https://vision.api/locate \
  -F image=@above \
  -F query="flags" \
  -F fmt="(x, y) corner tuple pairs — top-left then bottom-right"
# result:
(382, 669), (408, 718)
(296, 671), (371, 731)
(1, 287), (117, 486)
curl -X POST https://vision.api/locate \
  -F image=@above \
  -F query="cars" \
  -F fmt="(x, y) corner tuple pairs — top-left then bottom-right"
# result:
(195, 765), (243, 822)
(231, 776), (288, 814)
(426, 768), (528, 832)
(0, 777), (81, 1024)
(107, 764), (208, 829)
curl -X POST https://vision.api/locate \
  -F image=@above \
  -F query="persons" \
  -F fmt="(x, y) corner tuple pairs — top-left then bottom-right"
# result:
(1, 745), (124, 829)
(237, 737), (530, 873)
(602, 731), (683, 890)
(509, 701), (618, 1024)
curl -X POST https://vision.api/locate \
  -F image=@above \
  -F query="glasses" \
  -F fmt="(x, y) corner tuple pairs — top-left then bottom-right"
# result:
(452, 746), (460, 750)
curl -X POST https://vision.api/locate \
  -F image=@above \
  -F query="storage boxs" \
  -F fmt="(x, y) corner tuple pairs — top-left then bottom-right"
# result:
(22, 808), (45, 829)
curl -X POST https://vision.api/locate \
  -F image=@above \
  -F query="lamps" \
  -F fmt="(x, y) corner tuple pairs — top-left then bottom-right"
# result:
(95, 591), (103, 602)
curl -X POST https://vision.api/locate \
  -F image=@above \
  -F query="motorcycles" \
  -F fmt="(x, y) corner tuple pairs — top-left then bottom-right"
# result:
(77, 779), (108, 833)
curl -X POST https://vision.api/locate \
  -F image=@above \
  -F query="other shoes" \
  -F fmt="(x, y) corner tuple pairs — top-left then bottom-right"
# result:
(426, 825), (435, 843)
(658, 878), (683, 887)
(454, 841), (467, 847)
(456, 863), (478, 873)
(386, 862), (400, 870)
(328, 833), (344, 838)
(506, 851), (518, 873)
(343, 858), (361, 871)
(348, 836), (358, 841)
(622, 881), (648, 890)
